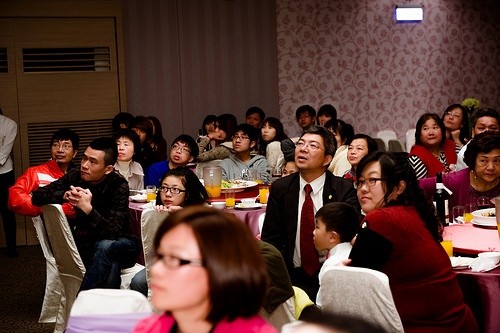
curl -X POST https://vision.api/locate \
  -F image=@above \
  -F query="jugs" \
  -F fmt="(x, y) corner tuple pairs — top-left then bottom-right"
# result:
(203, 166), (222, 197)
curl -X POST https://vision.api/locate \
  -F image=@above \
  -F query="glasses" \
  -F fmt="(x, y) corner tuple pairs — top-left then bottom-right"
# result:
(353, 176), (386, 188)
(296, 140), (328, 151)
(230, 134), (251, 140)
(158, 185), (185, 194)
(51, 143), (74, 150)
(148, 252), (206, 269)
(172, 144), (193, 152)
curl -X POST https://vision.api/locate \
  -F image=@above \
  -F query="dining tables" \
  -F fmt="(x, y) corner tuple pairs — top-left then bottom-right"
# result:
(442, 208), (500, 333)
(130, 178), (271, 266)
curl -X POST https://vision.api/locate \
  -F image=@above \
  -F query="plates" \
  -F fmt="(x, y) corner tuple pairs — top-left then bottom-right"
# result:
(449, 257), (473, 269)
(235, 203), (262, 208)
(221, 180), (258, 193)
(132, 198), (147, 202)
(470, 219), (497, 226)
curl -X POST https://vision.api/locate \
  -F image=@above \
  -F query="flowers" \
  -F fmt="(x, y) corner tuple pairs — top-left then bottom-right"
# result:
(461, 97), (480, 116)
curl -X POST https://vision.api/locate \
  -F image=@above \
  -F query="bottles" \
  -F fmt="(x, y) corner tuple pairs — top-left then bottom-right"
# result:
(432, 172), (450, 227)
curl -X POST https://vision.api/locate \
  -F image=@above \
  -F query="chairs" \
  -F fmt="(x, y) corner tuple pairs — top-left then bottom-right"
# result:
(32, 204), (176, 333)
(318, 266), (404, 333)
(368, 129), (417, 153)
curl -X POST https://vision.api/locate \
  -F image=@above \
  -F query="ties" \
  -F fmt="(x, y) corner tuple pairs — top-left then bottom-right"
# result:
(298, 184), (319, 277)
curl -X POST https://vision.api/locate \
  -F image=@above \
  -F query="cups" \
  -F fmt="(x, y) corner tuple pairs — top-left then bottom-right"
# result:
(259, 186), (269, 204)
(225, 190), (235, 209)
(464, 203), (476, 223)
(477, 198), (489, 210)
(252, 168), (262, 184)
(453, 206), (465, 224)
(272, 167), (282, 181)
(146, 186), (156, 202)
(439, 233), (453, 258)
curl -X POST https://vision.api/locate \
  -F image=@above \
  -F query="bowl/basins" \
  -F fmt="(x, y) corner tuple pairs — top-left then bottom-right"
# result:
(211, 202), (226, 209)
(478, 252), (500, 264)
(471, 208), (496, 224)
(241, 198), (255, 207)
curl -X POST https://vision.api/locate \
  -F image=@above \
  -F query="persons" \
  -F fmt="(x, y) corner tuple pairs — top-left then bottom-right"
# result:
(130, 206), (278, 333)
(8, 128), (80, 257)
(0, 114), (18, 259)
(31, 136), (142, 298)
(112, 103), (500, 303)
(340, 149), (481, 333)
